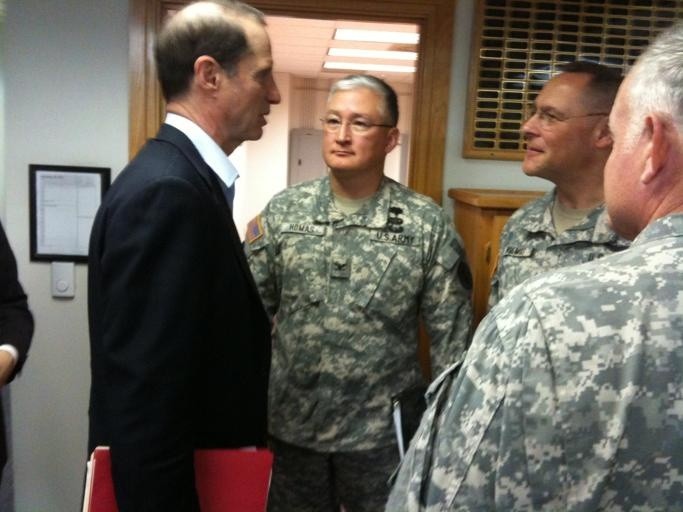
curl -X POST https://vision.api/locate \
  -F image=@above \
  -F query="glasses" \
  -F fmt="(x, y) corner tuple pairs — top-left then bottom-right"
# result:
(319, 114), (394, 136)
(525, 105), (609, 132)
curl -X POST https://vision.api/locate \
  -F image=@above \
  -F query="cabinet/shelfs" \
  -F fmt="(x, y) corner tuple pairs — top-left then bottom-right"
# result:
(448, 188), (555, 324)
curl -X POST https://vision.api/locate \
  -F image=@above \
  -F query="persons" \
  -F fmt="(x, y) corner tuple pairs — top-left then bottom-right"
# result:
(0, 220), (34, 480)
(487, 59), (637, 311)
(386, 19), (683, 511)
(244, 74), (472, 511)
(88, 1), (282, 512)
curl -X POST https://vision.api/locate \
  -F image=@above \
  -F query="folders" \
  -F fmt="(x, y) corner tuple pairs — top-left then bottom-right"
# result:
(392, 383), (428, 461)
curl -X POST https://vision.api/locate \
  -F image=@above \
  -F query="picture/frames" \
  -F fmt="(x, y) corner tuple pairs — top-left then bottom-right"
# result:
(27, 164), (112, 264)
